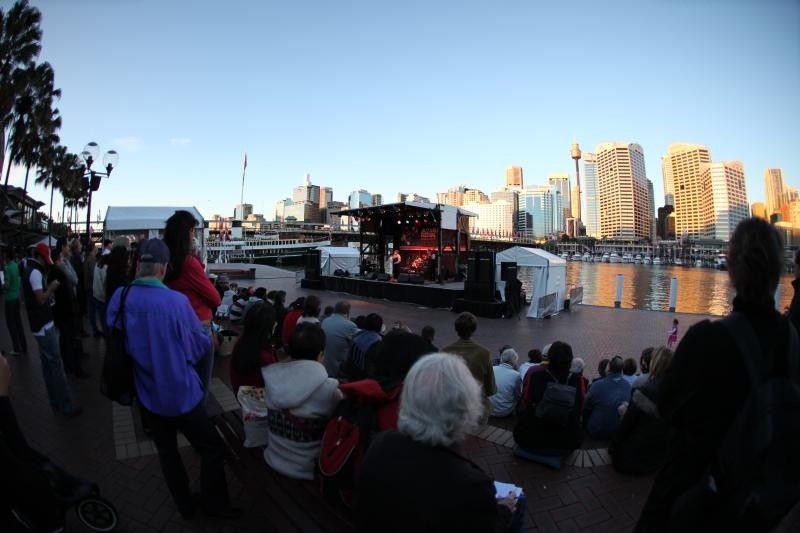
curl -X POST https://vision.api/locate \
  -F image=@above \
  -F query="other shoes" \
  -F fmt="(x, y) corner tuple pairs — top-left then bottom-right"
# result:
(389, 277), (398, 282)
(80, 370), (92, 379)
(8, 349), (20, 355)
(80, 352), (89, 359)
(93, 327), (102, 338)
(60, 404), (84, 417)
(81, 330), (90, 338)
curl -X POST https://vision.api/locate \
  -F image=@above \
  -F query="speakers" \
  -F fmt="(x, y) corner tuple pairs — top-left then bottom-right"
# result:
(463, 250), (496, 302)
(334, 269), (344, 276)
(376, 273), (390, 281)
(398, 273), (424, 284)
(365, 271), (377, 280)
(304, 250), (321, 280)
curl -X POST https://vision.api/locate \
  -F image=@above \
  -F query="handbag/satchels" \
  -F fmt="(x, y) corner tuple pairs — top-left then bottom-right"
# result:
(98, 325), (140, 407)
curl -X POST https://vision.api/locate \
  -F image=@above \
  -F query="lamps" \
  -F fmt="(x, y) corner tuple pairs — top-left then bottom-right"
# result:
(366, 208), (374, 215)
(392, 204), (401, 213)
(357, 210), (363, 216)
(379, 207), (385, 214)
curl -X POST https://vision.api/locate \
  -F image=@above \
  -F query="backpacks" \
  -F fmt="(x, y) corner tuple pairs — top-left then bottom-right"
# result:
(313, 385), (404, 490)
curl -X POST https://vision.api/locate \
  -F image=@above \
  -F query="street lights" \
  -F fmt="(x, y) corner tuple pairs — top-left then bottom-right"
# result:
(82, 142), (119, 240)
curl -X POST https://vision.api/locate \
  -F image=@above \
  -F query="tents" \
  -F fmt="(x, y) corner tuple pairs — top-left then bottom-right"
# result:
(101, 206), (207, 271)
(316, 247), (393, 274)
(495, 246), (568, 319)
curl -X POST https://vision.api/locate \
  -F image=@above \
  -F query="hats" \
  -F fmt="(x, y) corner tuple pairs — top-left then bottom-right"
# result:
(137, 238), (170, 263)
(541, 343), (553, 359)
(37, 243), (54, 265)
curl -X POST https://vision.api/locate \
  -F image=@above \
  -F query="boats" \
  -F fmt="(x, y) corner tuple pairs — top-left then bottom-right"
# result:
(206, 219), (333, 265)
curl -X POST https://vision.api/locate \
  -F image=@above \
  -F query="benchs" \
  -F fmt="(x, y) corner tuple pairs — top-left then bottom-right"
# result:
(202, 376), (366, 533)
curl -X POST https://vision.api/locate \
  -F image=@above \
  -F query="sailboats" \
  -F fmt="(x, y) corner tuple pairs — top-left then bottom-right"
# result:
(560, 241), (727, 267)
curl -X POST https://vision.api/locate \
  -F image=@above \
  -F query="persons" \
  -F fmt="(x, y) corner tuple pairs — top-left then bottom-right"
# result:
(0, 210), (800, 533)
